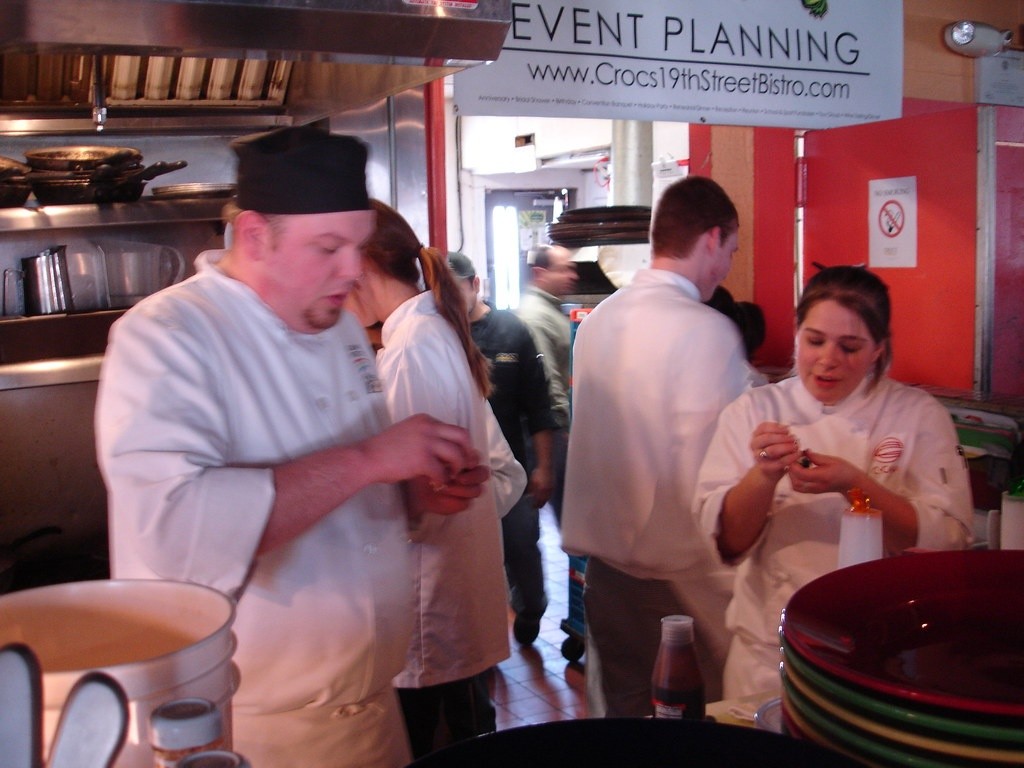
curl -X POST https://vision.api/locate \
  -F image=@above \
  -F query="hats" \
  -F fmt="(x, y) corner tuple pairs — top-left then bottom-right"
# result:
(229, 127), (370, 215)
(448, 251), (474, 279)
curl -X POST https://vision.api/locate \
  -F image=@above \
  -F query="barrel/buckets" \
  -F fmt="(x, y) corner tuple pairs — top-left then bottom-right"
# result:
(0, 578), (238, 768)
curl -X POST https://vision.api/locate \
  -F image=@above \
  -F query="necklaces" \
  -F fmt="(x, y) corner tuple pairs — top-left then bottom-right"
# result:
(758, 448), (767, 459)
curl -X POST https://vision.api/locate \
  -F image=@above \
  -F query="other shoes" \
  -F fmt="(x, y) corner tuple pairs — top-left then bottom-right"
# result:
(513, 613), (540, 644)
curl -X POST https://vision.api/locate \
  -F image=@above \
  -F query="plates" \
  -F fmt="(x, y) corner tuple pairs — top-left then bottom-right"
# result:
(781, 550), (1024, 768)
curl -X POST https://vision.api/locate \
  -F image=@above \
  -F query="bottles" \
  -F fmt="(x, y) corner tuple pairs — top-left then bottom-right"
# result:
(650, 616), (705, 719)
(177, 750), (248, 768)
(150, 698), (221, 768)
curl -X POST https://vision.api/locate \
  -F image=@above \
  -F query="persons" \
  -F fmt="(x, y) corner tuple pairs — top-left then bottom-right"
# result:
(445, 176), (768, 722)
(339, 198), (528, 759)
(93, 125), (491, 768)
(691, 265), (977, 734)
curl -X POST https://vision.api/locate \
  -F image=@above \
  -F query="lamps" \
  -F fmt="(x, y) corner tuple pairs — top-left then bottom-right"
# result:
(944, 19), (1024, 107)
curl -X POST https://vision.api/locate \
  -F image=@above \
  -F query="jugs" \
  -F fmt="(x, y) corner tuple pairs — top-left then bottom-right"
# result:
(97, 239), (185, 308)
(3, 245), (74, 316)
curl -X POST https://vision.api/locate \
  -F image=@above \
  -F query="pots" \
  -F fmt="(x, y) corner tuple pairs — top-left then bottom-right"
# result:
(27, 146), (187, 201)
